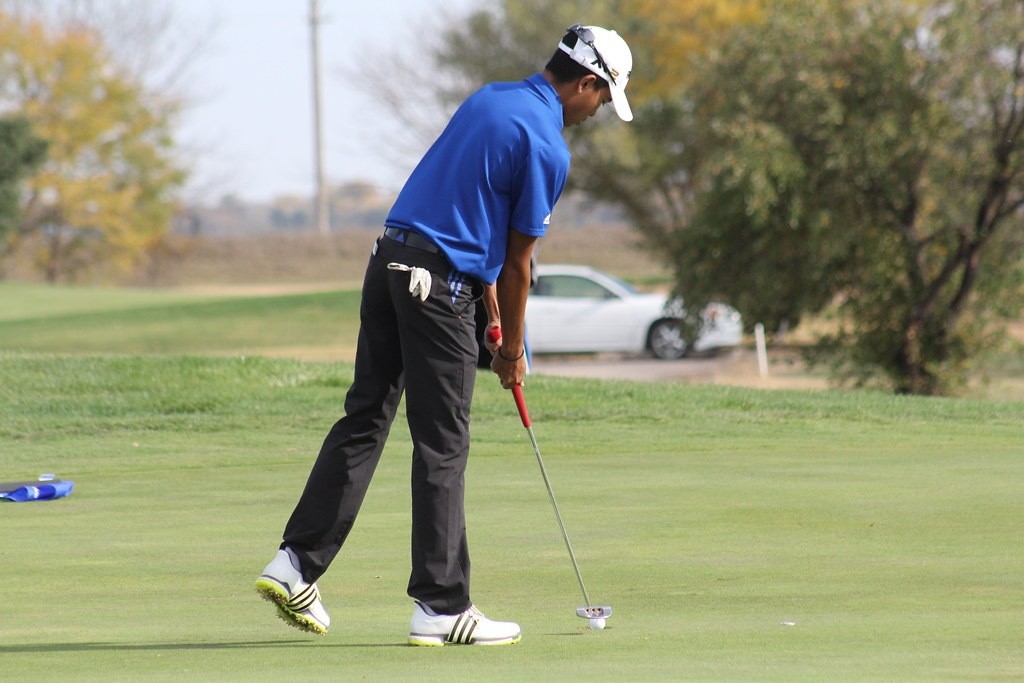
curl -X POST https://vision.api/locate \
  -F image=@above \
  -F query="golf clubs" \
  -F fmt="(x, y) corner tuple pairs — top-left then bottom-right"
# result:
(486, 325), (613, 619)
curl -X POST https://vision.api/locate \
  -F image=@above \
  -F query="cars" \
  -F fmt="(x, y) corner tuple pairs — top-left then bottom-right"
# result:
(493, 264), (746, 362)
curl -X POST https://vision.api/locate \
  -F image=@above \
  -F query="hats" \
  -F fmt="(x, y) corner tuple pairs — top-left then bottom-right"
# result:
(558, 23), (634, 122)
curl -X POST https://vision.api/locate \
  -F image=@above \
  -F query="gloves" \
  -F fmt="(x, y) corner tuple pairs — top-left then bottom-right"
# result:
(389, 262), (430, 302)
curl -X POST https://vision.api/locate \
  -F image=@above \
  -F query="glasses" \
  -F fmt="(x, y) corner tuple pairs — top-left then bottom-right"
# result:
(569, 23), (615, 85)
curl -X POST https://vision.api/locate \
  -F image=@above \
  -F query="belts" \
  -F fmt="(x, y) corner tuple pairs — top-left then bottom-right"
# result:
(384, 226), (440, 254)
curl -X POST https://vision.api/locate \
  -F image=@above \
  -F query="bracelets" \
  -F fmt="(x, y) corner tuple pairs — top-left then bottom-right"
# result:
(498, 347), (524, 361)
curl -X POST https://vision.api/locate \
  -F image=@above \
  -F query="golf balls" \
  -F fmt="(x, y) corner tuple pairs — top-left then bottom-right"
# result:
(589, 618), (606, 630)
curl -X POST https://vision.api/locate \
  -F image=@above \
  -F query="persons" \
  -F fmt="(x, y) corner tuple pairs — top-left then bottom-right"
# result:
(254, 22), (634, 646)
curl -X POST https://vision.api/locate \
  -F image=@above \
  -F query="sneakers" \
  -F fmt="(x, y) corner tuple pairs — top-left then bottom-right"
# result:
(409, 594), (521, 645)
(255, 549), (335, 629)
